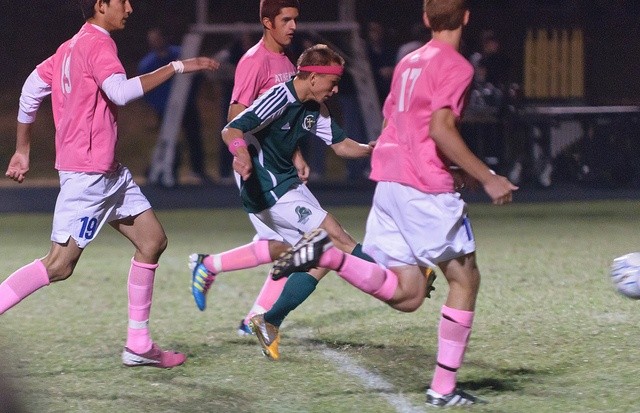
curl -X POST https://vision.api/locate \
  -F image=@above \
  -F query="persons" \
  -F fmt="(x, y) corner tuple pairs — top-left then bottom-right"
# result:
(187, 2), (310, 338)
(365, 20), (401, 97)
(206, 25), (244, 187)
(138, 21), (207, 187)
(305, 38), (368, 182)
(221, 44), (437, 362)
(273, 0), (518, 408)
(462, 33), (609, 187)
(1, 0), (220, 369)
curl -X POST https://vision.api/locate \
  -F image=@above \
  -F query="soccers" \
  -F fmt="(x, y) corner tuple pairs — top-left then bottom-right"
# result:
(611, 252), (640, 299)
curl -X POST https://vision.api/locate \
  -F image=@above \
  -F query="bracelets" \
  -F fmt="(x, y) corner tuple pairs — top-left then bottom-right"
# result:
(228, 138), (250, 152)
(168, 60), (184, 74)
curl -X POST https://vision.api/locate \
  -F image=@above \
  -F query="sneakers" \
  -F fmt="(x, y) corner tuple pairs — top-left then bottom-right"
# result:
(424, 388), (488, 408)
(122, 344), (187, 368)
(271, 228), (334, 281)
(248, 314), (281, 362)
(237, 318), (254, 336)
(188, 253), (214, 310)
(419, 266), (437, 298)
